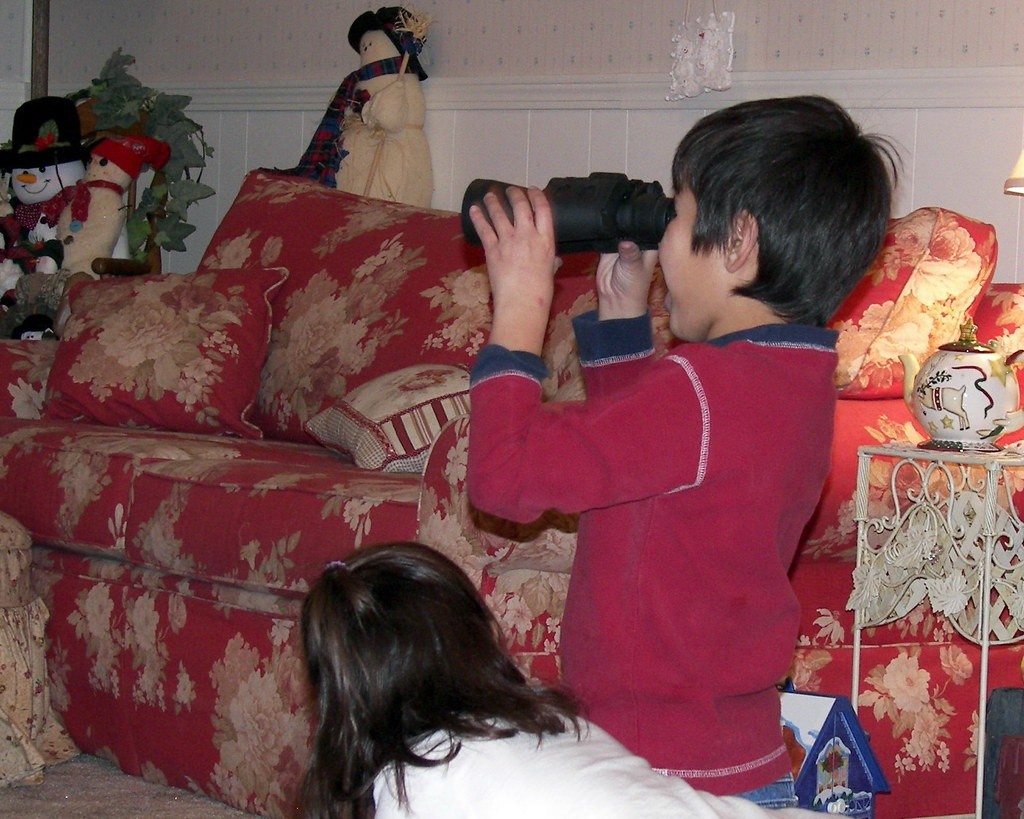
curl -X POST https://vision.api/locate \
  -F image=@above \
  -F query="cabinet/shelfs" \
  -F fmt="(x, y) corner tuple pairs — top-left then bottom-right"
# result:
(847, 441), (1024, 819)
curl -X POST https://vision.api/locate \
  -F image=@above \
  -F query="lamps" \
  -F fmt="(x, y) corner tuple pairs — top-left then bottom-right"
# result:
(1002, 149), (1024, 195)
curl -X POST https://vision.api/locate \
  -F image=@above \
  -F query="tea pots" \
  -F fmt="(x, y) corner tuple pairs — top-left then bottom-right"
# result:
(898, 316), (1024, 451)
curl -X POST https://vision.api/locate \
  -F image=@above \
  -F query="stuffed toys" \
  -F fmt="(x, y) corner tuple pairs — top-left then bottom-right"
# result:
(0, 90), (168, 342)
(331, 6), (432, 209)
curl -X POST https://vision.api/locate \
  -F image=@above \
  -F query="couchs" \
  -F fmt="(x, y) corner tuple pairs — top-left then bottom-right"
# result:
(0, 163), (1024, 819)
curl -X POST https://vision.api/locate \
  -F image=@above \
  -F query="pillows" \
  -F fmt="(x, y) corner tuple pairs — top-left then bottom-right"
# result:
(303, 360), (470, 468)
(43, 265), (288, 440)
(827, 206), (998, 410)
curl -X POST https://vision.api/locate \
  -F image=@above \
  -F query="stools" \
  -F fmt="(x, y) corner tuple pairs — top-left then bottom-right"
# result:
(0, 510), (80, 791)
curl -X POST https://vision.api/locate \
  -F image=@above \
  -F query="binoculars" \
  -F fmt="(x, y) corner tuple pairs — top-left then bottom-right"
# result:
(461, 172), (676, 251)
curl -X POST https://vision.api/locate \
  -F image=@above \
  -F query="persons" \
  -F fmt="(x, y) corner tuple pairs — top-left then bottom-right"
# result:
(466, 96), (911, 809)
(293, 541), (861, 819)
